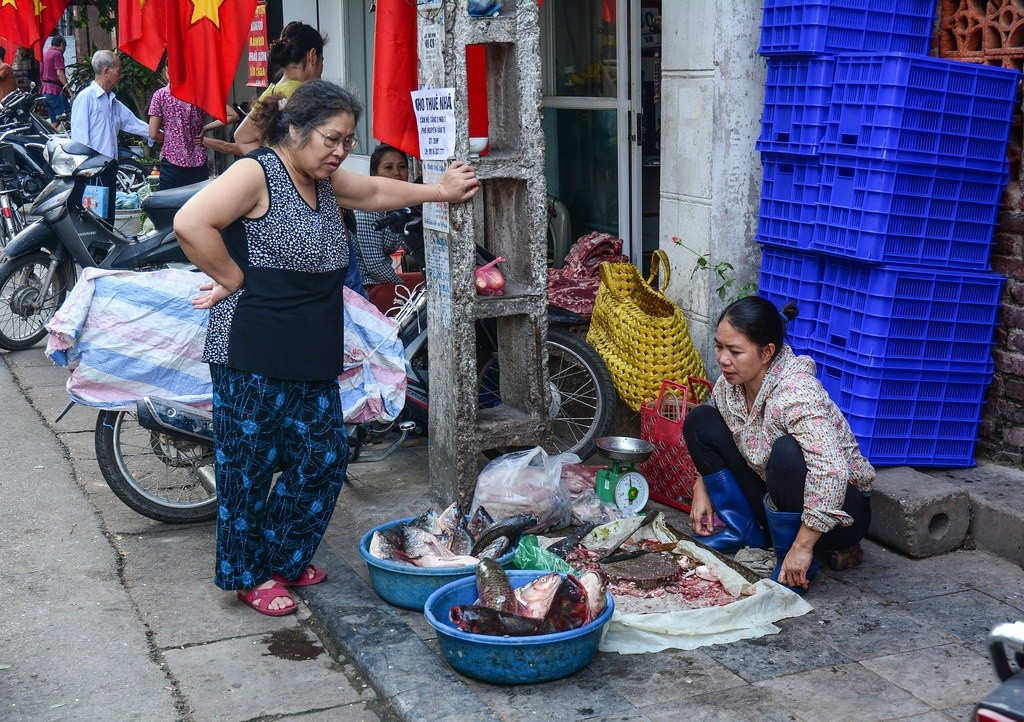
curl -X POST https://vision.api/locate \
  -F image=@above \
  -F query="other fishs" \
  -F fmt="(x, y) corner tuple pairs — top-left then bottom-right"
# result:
(370, 500), (540, 567)
(446, 555), (607, 636)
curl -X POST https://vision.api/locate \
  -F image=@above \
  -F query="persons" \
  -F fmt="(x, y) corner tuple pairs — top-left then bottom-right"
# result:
(202, 102), (251, 161)
(0, 46), (17, 105)
(40, 35), (74, 131)
(348, 143), (424, 314)
(69, 49), (164, 233)
(683, 296), (878, 597)
(233, 20), (369, 301)
(170, 79), (483, 616)
(148, 59), (238, 190)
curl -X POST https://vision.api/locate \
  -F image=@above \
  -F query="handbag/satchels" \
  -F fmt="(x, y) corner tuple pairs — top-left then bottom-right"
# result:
(82, 176), (109, 219)
(470, 445), (583, 534)
(585, 249), (709, 414)
(641, 377), (712, 514)
(474, 257), (507, 295)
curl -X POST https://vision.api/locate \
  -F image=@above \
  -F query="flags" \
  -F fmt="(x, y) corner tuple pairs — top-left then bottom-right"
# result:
(117, 0), (257, 123)
(0, 0), (71, 67)
(374, 0), (488, 159)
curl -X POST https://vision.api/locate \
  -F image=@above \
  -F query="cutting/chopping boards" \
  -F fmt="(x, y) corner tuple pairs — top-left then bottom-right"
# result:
(598, 551), (682, 589)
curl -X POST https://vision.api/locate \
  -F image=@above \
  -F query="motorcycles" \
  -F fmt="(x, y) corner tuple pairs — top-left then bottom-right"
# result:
(0, 82), (150, 217)
(0, 113), (228, 352)
(58, 199), (632, 511)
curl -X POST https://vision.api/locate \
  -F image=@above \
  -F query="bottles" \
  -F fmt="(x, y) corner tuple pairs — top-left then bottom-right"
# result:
(599, 32), (617, 97)
(147, 166), (160, 175)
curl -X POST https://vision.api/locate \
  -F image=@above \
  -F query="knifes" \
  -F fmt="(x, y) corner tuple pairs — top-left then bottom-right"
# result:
(595, 509), (660, 562)
(600, 543), (677, 563)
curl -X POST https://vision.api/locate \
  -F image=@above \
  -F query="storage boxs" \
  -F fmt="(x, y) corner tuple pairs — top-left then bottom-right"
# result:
(755, 0), (1024, 468)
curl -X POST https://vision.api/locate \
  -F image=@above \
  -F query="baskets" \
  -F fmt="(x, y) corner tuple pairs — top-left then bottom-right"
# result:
(647, 392), (698, 423)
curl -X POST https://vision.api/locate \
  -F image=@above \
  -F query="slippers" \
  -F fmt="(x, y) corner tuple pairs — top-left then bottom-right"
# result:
(236, 579), (298, 616)
(273, 563), (327, 586)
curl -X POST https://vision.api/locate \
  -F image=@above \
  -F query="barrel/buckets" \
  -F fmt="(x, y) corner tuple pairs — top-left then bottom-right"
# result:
(112, 208), (142, 239)
(145, 176), (159, 192)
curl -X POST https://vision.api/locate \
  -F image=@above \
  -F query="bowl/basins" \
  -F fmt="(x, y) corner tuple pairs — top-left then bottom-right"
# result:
(470, 138), (489, 157)
(360, 514), (615, 685)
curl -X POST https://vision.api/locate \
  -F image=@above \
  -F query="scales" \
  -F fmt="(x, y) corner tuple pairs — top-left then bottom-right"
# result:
(592, 437), (659, 516)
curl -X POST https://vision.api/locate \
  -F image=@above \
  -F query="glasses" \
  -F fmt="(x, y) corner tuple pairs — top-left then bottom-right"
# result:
(308, 123), (358, 152)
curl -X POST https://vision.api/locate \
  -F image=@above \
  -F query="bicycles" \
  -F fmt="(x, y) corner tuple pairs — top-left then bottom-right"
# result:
(0, 127), (37, 278)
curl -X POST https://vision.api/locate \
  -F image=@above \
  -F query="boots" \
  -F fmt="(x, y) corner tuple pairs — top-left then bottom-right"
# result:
(762, 492), (823, 596)
(694, 469), (768, 554)
